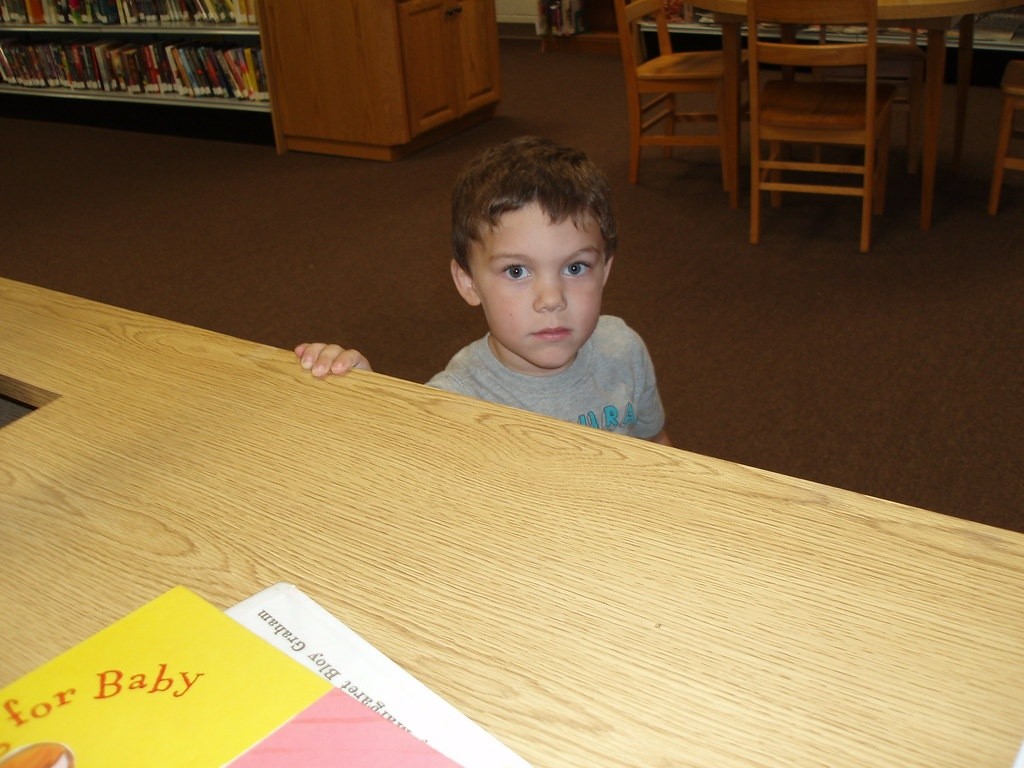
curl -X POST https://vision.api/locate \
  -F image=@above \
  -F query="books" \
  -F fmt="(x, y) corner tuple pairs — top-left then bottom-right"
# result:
(0, 0), (257, 26)
(0, 586), (466, 767)
(544, 0), (585, 36)
(0, 40), (271, 102)
(699, 11), (1021, 43)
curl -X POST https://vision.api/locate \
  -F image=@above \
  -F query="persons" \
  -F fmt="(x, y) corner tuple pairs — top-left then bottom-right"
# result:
(293, 135), (673, 449)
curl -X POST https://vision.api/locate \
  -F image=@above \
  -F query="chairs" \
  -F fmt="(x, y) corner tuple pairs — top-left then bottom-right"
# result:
(747, 0), (927, 255)
(987, 60), (1024, 216)
(614, 0), (749, 192)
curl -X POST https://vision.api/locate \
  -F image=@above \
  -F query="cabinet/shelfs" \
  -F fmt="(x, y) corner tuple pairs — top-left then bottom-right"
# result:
(0, 22), (277, 148)
(252, 0), (503, 164)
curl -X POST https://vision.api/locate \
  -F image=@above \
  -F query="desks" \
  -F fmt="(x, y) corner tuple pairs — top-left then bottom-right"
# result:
(687, 0), (1024, 237)
